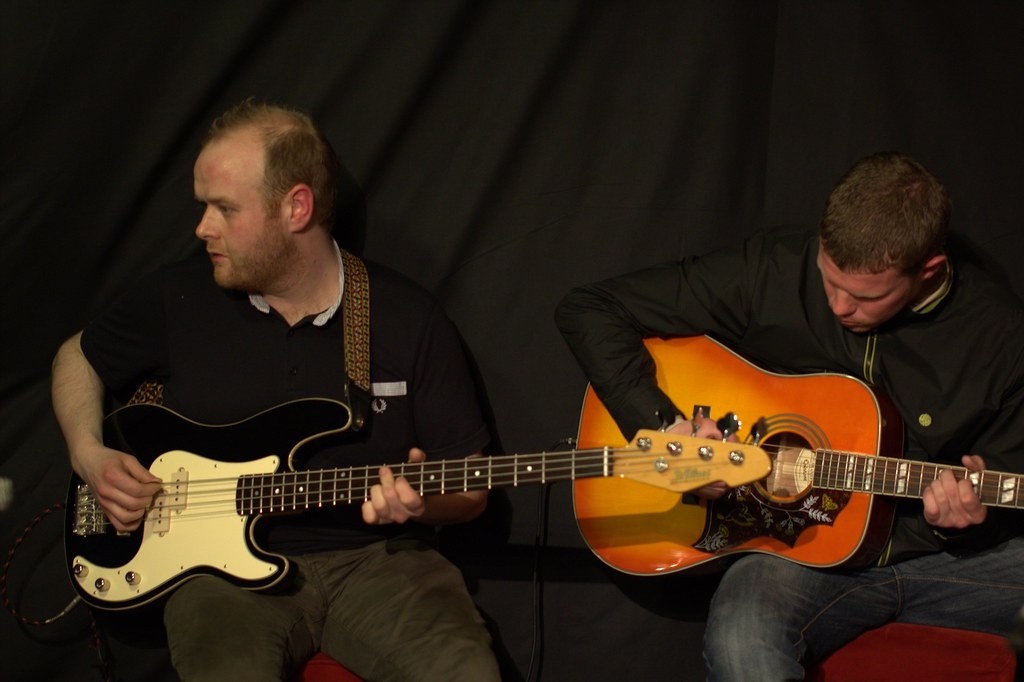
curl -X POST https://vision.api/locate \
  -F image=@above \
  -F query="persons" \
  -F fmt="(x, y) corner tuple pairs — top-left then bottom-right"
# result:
(50, 97), (500, 682)
(552, 151), (1024, 682)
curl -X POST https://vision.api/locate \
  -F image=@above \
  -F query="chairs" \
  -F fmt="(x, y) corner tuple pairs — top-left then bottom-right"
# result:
(809, 621), (1018, 682)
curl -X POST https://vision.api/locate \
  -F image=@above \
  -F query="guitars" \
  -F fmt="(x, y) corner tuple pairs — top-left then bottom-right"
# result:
(60, 394), (772, 618)
(569, 331), (1023, 587)
